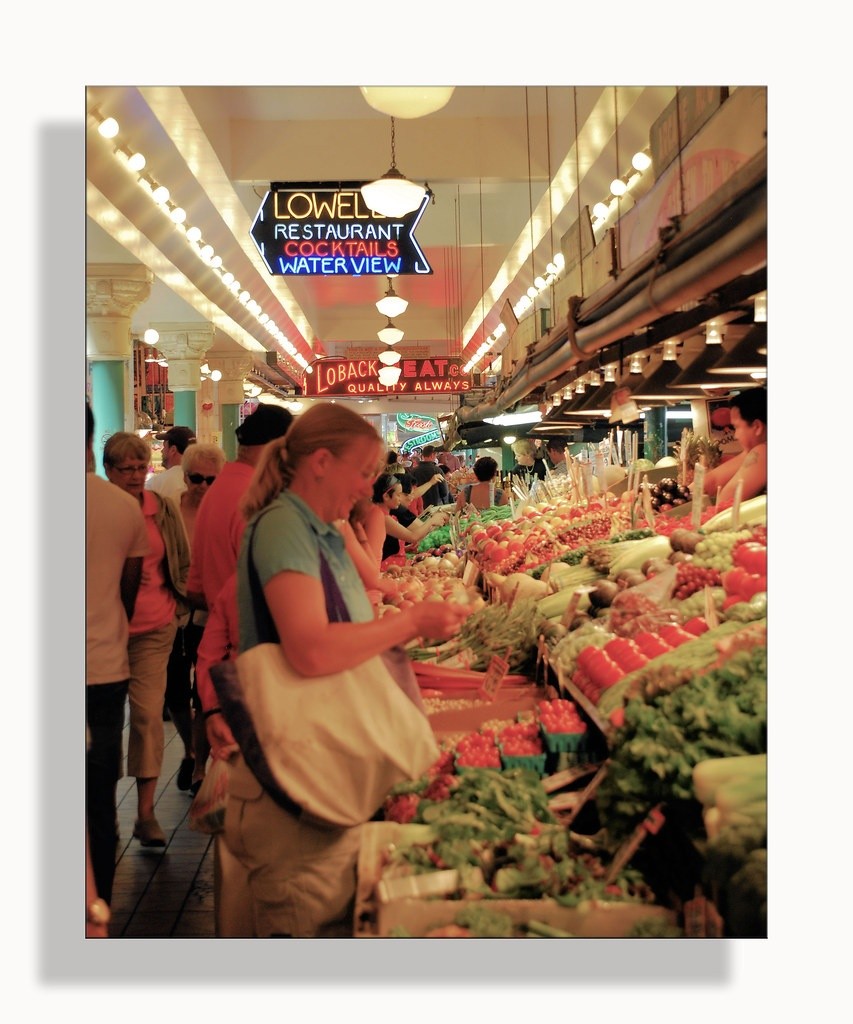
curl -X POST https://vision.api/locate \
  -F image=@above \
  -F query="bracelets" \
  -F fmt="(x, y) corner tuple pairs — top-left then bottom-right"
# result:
(203, 706), (222, 721)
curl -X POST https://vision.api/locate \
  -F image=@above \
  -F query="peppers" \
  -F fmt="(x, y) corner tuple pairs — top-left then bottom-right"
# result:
(388, 769), (655, 937)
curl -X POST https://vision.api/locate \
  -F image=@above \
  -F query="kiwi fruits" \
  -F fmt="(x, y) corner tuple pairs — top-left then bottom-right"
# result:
(670, 528), (686, 550)
(642, 558), (669, 571)
(617, 568), (639, 579)
(629, 574), (646, 586)
(588, 580), (618, 607)
(573, 608), (593, 624)
(681, 534), (703, 552)
(650, 564), (669, 578)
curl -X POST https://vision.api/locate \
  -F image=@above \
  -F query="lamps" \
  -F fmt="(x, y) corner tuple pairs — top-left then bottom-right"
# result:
(552, 372), (600, 407)
(754, 290), (767, 322)
(145, 349), (155, 362)
(706, 321), (723, 344)
(361, 115), (425, 218)
(377, 317), (405, 345)
(359, 87), (456, 119)
(663, 338), (677, 361)
(604, 364), (615, 382)
(375, 277), (409, 318)
(155, 350), (169, 368)
(377, 344), (402, 365)
(630, 353), (642, 373)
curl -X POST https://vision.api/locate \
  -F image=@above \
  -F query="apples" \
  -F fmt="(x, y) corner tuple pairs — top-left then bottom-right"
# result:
(462, 491), (637, 554)
(373, 542), (484, 620)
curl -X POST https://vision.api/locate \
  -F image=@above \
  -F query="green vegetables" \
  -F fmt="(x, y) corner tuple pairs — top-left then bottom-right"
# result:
(597, 647), (767, 853)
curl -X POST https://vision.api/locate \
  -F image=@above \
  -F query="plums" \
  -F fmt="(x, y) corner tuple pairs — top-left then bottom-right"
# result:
(638, 477), (690, 512)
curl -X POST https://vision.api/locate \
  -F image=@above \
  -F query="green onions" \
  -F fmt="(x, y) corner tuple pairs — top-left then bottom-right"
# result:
(409, 599), (551, 670)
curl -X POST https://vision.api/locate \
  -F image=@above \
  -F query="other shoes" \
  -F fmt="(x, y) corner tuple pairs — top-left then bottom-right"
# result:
(191, 778), (203, 797)
(177, 755), (197, 791)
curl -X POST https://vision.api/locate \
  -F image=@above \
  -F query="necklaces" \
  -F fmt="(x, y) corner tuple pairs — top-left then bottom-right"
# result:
(526, 461), (535, 473)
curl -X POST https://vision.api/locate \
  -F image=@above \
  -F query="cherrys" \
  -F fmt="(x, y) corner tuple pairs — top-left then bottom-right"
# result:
(496, 517), (612, 576)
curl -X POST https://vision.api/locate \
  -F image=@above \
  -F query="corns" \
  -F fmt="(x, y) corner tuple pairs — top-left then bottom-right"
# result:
(531, 536), (673, 623)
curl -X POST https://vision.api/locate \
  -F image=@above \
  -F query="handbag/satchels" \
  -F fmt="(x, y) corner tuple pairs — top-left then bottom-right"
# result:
(208, 504), (441, 827)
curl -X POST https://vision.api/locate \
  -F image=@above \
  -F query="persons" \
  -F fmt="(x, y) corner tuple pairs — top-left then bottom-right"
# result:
(703, 384), (768, 506)
(86, 402), (152, 906)
(87, 828), (112, 938)
(183, 404), (389, 759)
(370, 443), (456, 560)
(101, 423), (229, 849)
(510, 439), (546, 490)
(219, 400), (478, 936)
(548, 438), (574, 476)
(456, 457), (510, 510)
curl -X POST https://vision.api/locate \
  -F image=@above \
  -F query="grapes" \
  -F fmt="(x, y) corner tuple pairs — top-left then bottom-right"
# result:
(674, 528), (753, 599)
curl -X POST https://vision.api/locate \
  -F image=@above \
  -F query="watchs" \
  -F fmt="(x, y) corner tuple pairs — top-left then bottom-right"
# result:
(89, 899), (111, 926)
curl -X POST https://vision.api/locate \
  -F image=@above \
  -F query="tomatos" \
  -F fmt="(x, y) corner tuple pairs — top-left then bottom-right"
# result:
(383, 699), (588, 823)
(570, 617), (707, 706)
(722, 531), (767, 609)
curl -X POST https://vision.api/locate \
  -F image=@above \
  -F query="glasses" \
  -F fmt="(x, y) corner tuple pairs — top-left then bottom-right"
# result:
(186, 471), (217, 485)
(110, 464), (150, 474)
(710, 422), (736, 431)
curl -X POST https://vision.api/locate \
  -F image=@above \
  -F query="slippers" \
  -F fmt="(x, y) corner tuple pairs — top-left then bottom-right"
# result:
(132, 819), (168, 847)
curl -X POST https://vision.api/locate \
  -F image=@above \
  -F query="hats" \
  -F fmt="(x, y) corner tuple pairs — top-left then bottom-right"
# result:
(155, 426), (197, 448)
(234, 403), (293, 446)
(545, 437), (569, 453)
